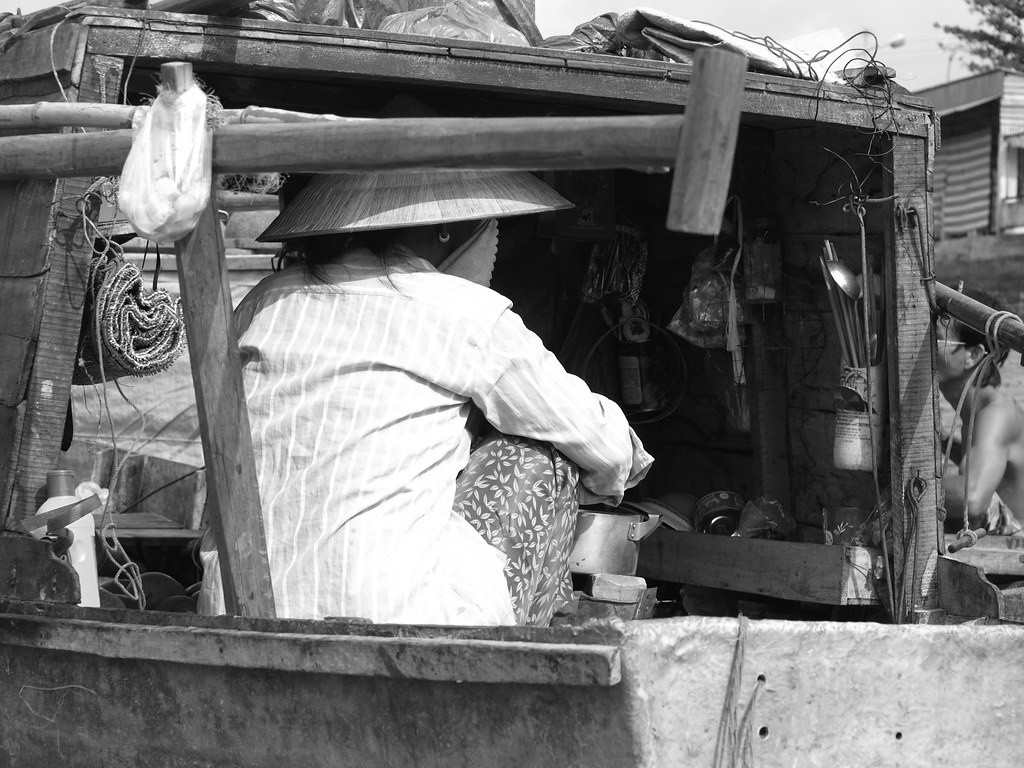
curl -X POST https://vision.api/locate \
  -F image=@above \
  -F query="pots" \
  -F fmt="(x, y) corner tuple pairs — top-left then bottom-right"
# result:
(567, 509), (663, 576)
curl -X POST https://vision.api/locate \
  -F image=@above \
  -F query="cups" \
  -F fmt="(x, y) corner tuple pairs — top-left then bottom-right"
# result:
(823, 507), (874, 546)
(615, 339), (663, 413)
(833, 344), (886, 472)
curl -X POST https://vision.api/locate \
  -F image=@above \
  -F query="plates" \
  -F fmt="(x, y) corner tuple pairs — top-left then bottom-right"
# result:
(115, 594), (139, 609)
(154, 596), (198, 614)
(621, 501), (693, 531)
(127, 572), (184, 610)
(100, 579), (130, 595)
(98, 587), (126, 609)
(186, 581), (202, 599)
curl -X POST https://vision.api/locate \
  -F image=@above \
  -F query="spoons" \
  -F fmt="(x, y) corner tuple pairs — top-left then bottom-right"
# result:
(623, 318), (650, 342)
(827, 260), (864, 368)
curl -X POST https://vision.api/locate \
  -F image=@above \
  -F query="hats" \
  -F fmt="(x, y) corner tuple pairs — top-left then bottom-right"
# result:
(255, 171), (576, 243)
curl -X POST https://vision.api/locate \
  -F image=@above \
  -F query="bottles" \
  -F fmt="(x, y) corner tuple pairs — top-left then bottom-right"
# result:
(33, 471), (100, 607)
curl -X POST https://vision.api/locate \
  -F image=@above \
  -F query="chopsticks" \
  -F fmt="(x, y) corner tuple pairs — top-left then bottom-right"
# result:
(818, 239), (885, 367)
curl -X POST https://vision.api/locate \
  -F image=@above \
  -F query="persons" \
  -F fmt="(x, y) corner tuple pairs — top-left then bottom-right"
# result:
(196, 166), (656, 629)
(936, 286), (1024, 531)
